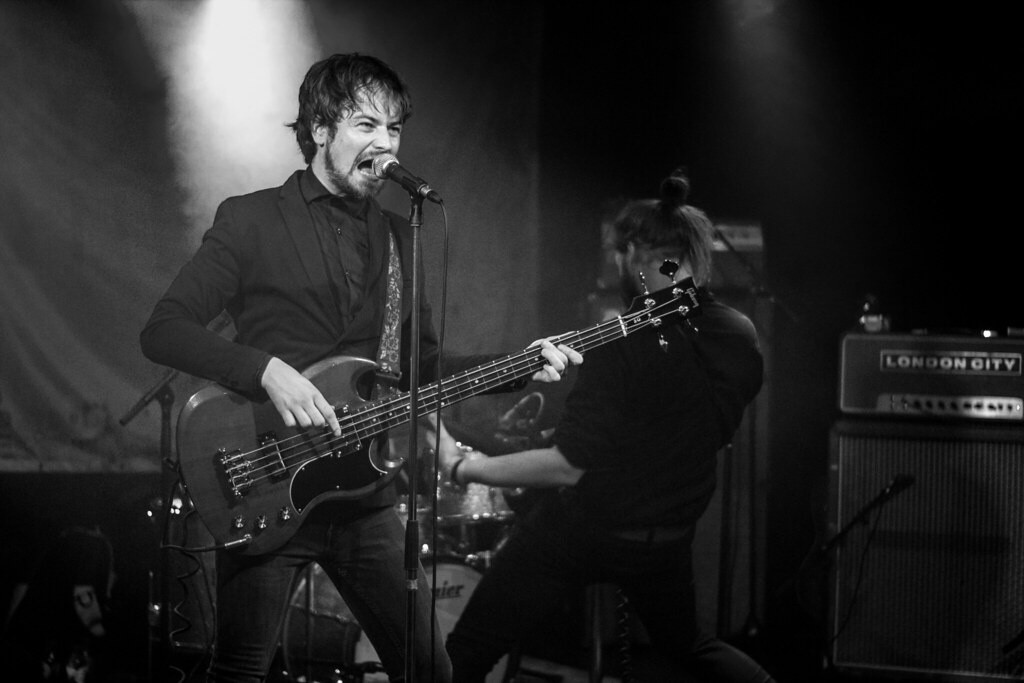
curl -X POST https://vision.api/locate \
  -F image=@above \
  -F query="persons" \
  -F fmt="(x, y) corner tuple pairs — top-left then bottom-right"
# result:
(445, 174), (764, 682)
(142, 53), (584, 683)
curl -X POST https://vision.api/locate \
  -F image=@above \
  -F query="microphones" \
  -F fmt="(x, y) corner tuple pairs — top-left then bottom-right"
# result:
(499, 392), (537, 431)
(369, 153), (443, 202)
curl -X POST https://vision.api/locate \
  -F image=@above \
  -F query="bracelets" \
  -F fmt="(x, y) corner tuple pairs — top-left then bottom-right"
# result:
(447, 450), (466, 492)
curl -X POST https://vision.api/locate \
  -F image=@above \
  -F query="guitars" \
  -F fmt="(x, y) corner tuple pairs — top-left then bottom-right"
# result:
(417, 412), (568, 504)
(173, 260), (703, 559)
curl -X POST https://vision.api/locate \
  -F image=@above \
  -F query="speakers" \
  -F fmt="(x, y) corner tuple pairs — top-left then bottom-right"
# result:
(822, 421), (1024, 683)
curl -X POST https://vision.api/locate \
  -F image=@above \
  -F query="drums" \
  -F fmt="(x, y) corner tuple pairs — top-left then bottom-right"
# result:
(392, 491), (433, 557)
(279, 543), (525, 683)
(422, 442), (520, 529)
